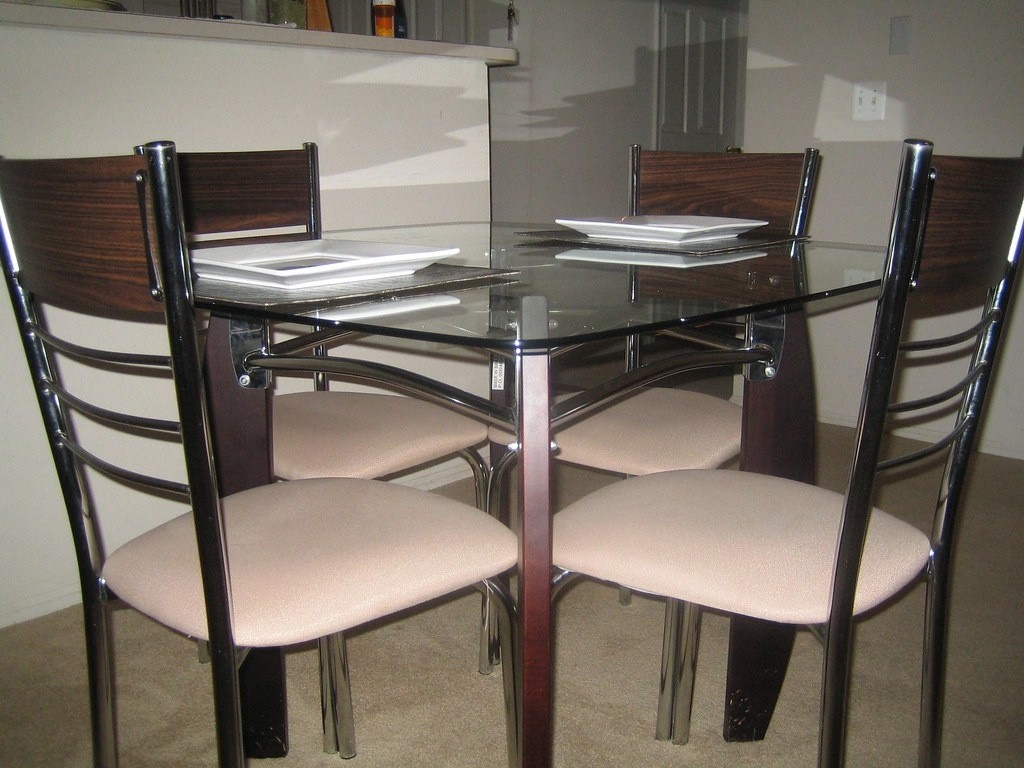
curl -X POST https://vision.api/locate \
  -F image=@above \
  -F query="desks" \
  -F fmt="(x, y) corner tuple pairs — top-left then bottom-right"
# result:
(184, 220), (890, 768)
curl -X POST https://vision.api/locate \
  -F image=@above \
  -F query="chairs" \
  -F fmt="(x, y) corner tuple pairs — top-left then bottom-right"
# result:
(133, 142), (551, 752)
(552, 140), (1024, 768)
(0, 142), (525, 767)
(494, 142), (821, 741)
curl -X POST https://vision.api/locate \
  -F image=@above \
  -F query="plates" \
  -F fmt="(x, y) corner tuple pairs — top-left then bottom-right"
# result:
(554, 248), (768, 268)
(188, 238), (460, 289)
(554, 214), (769, 243)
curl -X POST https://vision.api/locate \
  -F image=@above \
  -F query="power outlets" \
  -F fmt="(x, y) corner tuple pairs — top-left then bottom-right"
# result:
(852, 80), (887, 123)
